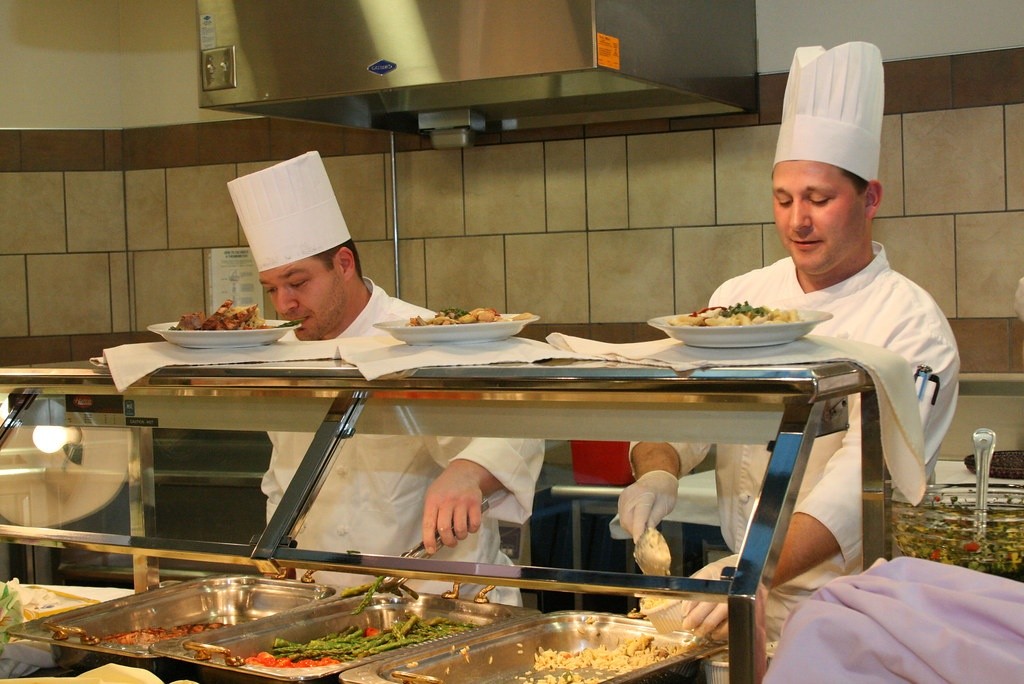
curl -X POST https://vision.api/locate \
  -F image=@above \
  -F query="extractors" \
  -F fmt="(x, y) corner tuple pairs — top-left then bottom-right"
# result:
(196, 0), (758, 149)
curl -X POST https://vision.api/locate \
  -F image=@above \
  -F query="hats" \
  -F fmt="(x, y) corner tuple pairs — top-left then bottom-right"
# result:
(228, 149), (351, 273)
(771, 41), (885, 182)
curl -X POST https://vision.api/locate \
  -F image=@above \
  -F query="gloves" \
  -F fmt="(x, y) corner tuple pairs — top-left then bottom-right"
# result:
(683, 552), (729, 645)
(617, 469), (679, 544)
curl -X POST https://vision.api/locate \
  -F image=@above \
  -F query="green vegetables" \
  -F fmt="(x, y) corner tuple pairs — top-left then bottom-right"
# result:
(720, 301), (767, 319)
(439, 308), (469, 319)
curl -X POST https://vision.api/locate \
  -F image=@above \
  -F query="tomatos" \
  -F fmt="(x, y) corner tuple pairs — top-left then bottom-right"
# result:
(242, 652), (340, 669)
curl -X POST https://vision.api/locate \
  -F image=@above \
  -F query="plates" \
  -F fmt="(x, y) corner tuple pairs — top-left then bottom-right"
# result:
(646, 309), (833, 348)
(146, 320), (299, 348)
(372, 313), (541, 346)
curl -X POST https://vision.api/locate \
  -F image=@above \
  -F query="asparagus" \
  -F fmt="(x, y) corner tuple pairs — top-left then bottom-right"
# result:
(269, 573), (484, 662)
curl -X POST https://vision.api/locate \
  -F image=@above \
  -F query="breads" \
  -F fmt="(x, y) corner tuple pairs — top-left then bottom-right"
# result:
(171, 299), (276, 330)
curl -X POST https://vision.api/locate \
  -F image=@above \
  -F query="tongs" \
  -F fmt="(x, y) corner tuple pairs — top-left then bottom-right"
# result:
(377, 490), (510, 591)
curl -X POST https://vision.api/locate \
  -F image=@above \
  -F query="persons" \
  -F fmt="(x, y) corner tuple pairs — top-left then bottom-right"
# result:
(227, 151), (545, 609)
(610, 41), (960, 643)
(1014, 277), (1024, 322)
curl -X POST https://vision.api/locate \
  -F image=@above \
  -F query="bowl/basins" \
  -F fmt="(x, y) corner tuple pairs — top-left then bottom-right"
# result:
(640, 598), (685, 634)
(704, 652), (730, 684)
